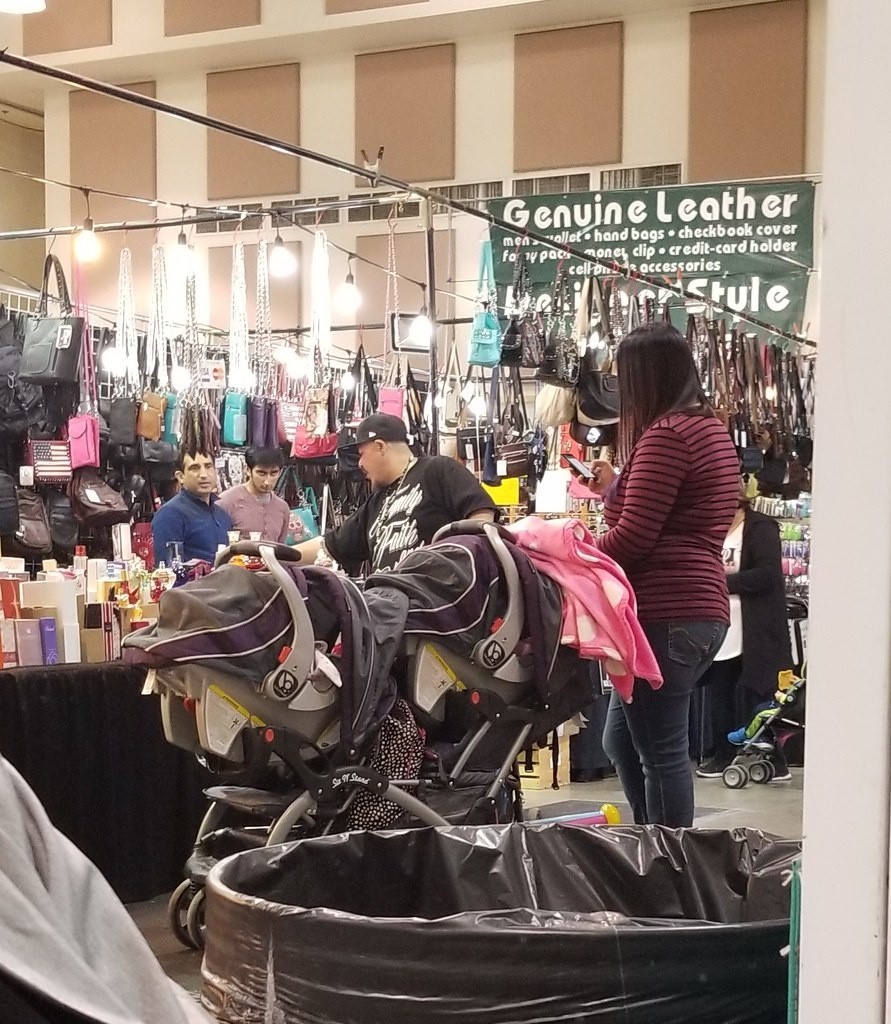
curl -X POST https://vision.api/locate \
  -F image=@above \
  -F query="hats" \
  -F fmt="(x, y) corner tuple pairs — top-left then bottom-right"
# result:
(337, 412), (406, 450)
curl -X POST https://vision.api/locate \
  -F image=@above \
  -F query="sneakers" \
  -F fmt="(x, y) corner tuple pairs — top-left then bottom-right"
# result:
(695, 752), (739, 778)
(727, 727), (748, 746)
(770, 760), (792, 781)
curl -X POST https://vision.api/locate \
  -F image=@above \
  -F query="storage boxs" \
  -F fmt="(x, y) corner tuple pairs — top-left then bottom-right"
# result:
(0, 523), (159, 669)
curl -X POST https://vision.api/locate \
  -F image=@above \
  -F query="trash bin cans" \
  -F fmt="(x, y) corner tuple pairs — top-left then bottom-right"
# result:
(197, 823), (802, 1024)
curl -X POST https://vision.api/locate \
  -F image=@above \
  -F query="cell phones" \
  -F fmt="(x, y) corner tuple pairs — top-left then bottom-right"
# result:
(560, 453), (599, 481)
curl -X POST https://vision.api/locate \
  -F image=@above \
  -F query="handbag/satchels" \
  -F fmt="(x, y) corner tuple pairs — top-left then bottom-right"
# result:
(167, 391), (287, 461)
(136, 391), (167, 442)
(140, 437), (173, 465)
(467, 313), (503, 368)
(98, 468), (173, 523)
(534, 337), (580, 388)
(713, 409), (812, 495)
(285, 505), (319, 545)
(576, 371), (621, 427)
(500, 313), (547, 368)
(107, 397), (136, 446)
(455, 423), (544, 479)
(26, 440), (73, 485)
(17, 252), (86, 386)
(58, 414), (100, 469)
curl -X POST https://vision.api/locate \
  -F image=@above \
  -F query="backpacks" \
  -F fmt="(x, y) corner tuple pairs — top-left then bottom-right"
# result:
(79, 519), (111, 561)
(66, 467), (129, 525)
(0, 471), (20, 536)
(15, 489), (53, 555)
(0, 346), (46, 438)
(105, 446), (140, 467)
(132, 523), (155, 573)
(46, 492), (79, 548)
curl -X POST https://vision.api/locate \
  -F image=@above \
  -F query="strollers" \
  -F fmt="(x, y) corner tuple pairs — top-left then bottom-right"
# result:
(124, 519), (597, 951)
(723, 659), (805, 789)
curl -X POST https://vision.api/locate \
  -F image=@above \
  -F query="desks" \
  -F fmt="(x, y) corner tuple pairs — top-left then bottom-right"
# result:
(0, 658), (342, 906)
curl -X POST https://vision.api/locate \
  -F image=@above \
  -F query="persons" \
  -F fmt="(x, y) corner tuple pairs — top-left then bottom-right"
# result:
(150, 450), (234, 588)
(695, 491), (795, 782)
(287, 411), (501, 579)
(214, 446), (290, 544)
(569, 322), (739, 828)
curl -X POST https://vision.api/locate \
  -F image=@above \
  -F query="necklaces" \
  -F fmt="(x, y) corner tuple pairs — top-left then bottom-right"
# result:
(376, 455), (414, 537)
